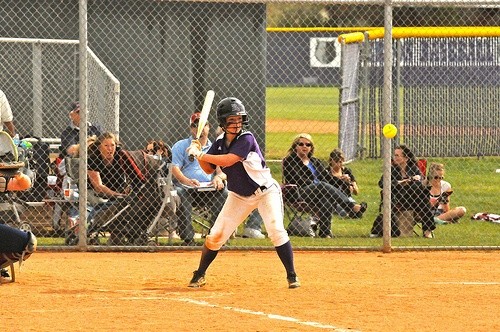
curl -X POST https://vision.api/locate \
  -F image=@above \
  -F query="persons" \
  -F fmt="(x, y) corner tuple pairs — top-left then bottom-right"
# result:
(423, 160), (466, 224)
(370, 146), (437, 239)
(0, 224), (38, 284)
(184, 97), (301, 289)
(326, 147), (358, 197)
(280, 132), (367, 239)
(172, 113), (233, 245)
(146, 139), (201, 219)
(57, 101), (100, 166)
(0, 88), (15, 138)
(243, 209), (266, 240)
(67, 130), (155, 244)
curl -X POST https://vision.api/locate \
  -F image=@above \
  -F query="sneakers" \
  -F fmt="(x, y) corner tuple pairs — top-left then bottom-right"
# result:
(188, 271), (206, 287)
(287, 272), (300, 287)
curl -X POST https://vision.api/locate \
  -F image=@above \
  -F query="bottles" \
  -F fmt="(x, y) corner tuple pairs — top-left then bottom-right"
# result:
(61, 176), (71, 201)
(20, 141), (32, 148)
(13, 134), (20, 148)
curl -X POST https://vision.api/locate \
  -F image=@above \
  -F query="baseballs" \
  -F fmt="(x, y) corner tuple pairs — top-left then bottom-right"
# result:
(383, 124), (397, 138)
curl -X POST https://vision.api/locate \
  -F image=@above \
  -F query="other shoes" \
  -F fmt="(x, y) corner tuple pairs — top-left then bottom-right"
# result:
(0, 268), (12, 283)
(243, 228), (265, 238)
(370, 234), (378, 237)
(183, 239), (194, 246)
(354, 202), (367, 218)
(320, 235), (334, 238)
(424, 231), (435, 238)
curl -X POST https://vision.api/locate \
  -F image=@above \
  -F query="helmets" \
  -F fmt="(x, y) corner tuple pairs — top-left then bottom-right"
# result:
(190, 113), (207, 127)
(216, 97), (247, 123)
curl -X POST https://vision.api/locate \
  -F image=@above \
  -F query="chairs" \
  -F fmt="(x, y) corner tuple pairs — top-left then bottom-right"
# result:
(283, 182), (320, 230)
(0, 131), (25, 222)
(380, 159), (432, 237)
(67, 149), (213, 245)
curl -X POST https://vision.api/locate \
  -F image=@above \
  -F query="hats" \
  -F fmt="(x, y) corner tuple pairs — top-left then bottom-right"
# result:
(71, 101), (80, 111)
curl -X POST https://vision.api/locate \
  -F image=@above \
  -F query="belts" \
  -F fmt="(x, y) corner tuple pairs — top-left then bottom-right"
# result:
(253, 186), (265, 194)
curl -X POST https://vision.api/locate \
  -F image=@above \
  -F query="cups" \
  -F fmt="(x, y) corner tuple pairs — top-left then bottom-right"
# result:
(47, 176), (57, 186)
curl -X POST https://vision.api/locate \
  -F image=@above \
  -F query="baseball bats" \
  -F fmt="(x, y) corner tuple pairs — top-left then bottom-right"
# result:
(189, 90), (215, 162)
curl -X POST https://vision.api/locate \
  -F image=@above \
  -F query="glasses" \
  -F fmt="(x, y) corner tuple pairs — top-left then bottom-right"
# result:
(435, 176), (443, 179)
(297, 142), (311, 147)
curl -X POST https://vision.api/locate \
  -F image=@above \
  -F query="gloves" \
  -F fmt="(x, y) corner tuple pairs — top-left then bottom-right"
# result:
(186, 140), (205, 161)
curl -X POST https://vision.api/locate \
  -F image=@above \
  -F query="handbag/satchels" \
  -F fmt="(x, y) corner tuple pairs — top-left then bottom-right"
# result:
(289, 215), (319, 238)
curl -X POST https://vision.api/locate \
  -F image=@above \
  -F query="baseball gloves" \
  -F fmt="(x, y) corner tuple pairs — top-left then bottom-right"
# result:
(0, 161), (25, 178)
